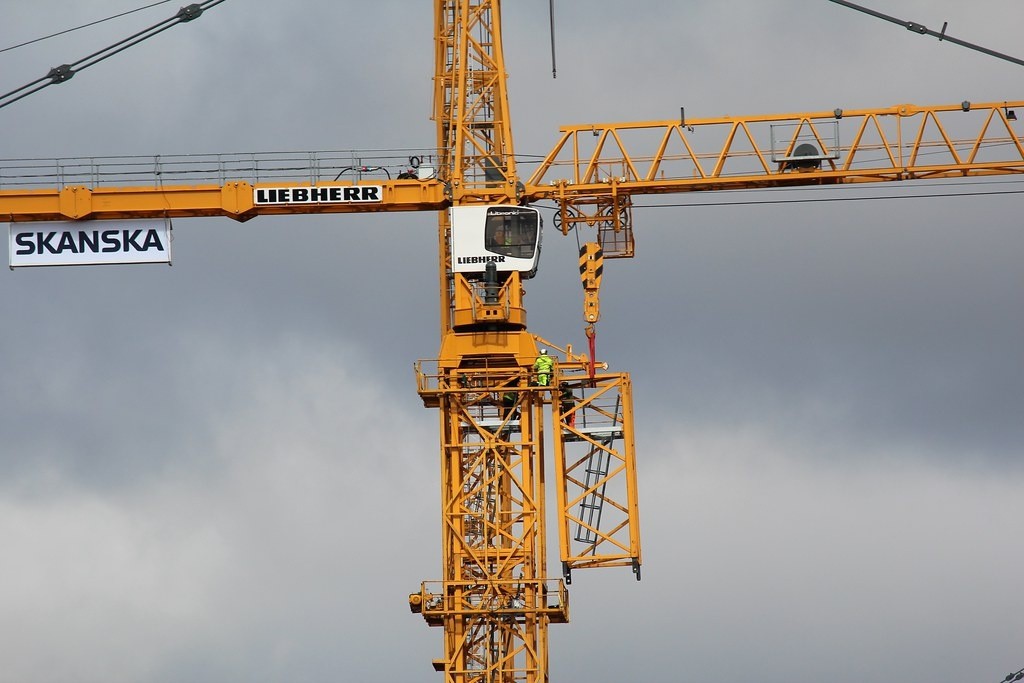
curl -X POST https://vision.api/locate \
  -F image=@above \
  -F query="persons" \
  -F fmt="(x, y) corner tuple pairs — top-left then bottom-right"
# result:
(559, 382), (575, 429)
(533, 349), (554, 386)
(503, 392), (516, 420)
(504, 225), (512, 248)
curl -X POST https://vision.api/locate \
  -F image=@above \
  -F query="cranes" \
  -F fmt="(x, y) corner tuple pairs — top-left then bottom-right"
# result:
(0, 0), (1023, 682)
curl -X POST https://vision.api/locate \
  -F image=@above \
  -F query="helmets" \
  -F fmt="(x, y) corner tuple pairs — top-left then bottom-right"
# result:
(540, 348), (547, 354)
